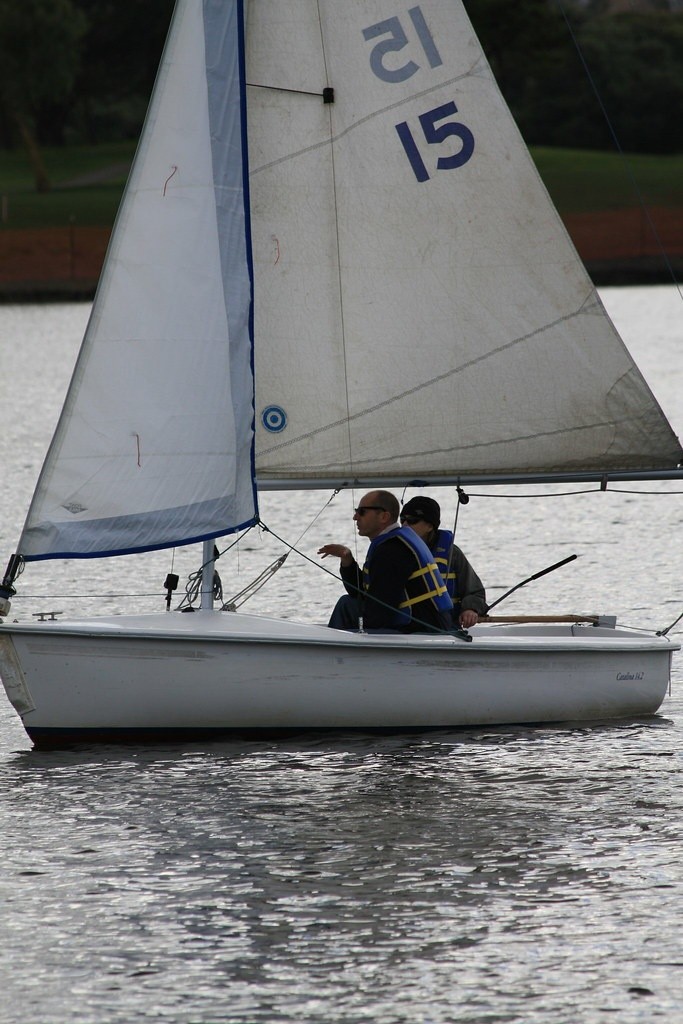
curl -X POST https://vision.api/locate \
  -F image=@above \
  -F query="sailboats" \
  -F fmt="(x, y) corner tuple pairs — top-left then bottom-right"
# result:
(0, 0), (683, 749)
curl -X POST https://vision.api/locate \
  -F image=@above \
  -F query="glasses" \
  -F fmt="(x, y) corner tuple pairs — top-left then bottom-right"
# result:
(354, 506), (387, 516)
(400, 514), (422, 525)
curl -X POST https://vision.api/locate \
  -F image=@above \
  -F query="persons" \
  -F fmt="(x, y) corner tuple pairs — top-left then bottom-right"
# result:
(399, 496), (487, 629)
(317, 489), (456, 635)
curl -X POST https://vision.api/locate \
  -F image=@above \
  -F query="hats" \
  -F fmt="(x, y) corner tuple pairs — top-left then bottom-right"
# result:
(399, 495), (441, 530)
